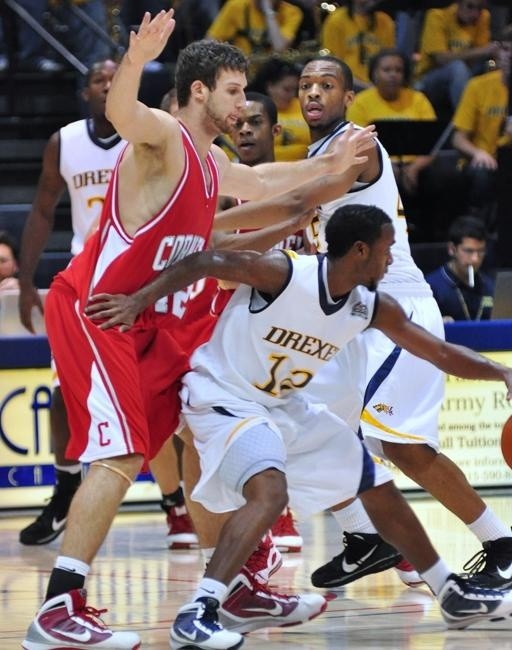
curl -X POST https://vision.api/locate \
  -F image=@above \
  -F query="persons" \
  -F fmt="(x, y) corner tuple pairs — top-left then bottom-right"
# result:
(1, 232), (18, 290)
(160, 0), (512, 587)
(19, 8), (377, 650)
(84, 205), (510, 648)
(18, 60), (185, 545)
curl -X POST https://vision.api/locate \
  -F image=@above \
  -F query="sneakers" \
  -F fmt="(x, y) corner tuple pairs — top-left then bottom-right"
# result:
(434, 526), (512, 631)
(20, 589), (141, 650)
(19, 467), (82, 545)
(312, 530), (403, 588)
(395, 557), (427, 587)
(160, 496), (328, 649)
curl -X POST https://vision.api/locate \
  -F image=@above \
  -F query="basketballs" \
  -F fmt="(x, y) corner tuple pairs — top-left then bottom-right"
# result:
(501, 415), (511, 468)
